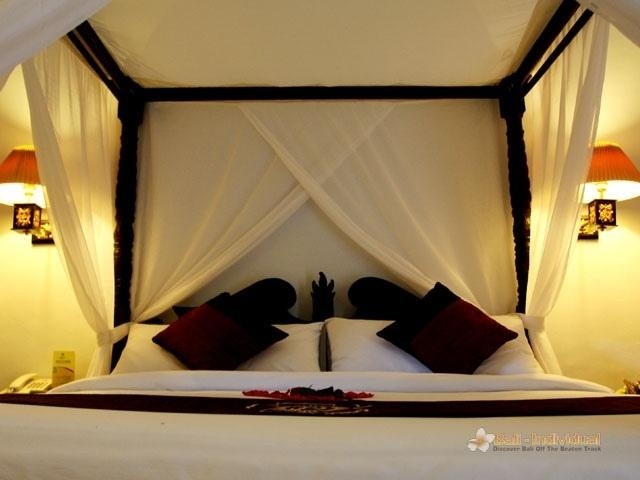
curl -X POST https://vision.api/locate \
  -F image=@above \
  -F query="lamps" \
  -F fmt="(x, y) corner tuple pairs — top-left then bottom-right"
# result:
(0, 144), (54, 245)
(576, 142), (639, 240)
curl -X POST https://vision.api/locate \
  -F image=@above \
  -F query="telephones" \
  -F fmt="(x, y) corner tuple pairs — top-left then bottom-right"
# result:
(10, 373), (52, 394)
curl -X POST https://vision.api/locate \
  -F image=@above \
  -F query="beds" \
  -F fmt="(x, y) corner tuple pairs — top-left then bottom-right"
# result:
(0, 80), (639, 478)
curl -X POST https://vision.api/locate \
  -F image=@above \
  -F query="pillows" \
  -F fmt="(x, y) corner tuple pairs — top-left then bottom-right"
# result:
(108, 281), (544, 373)
